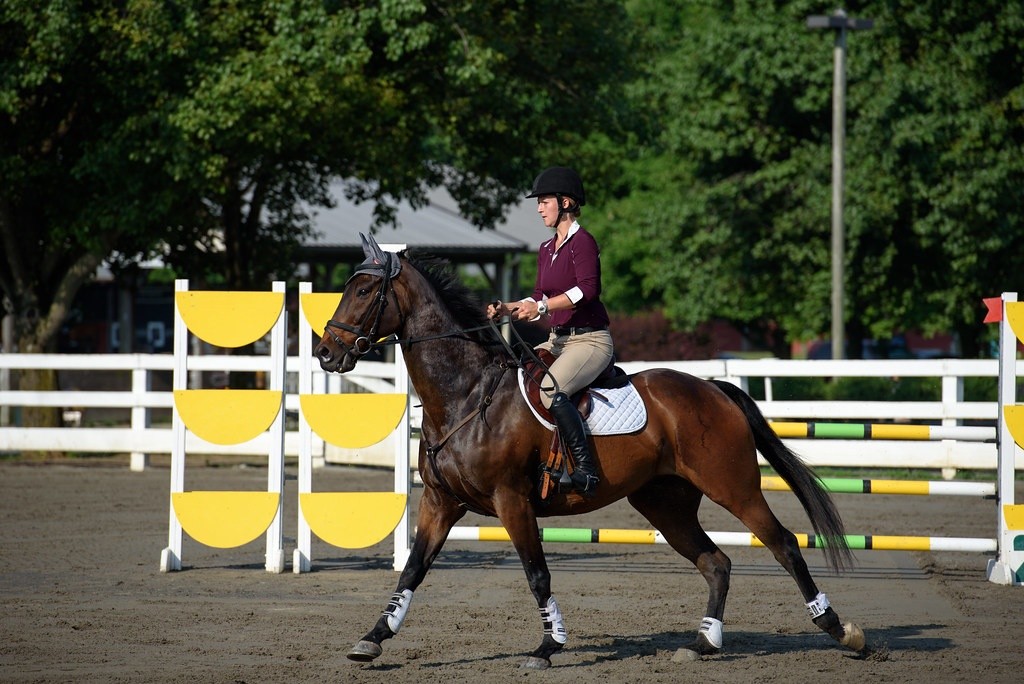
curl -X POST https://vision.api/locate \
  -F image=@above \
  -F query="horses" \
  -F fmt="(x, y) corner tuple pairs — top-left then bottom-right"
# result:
(315, 231), (864, 669)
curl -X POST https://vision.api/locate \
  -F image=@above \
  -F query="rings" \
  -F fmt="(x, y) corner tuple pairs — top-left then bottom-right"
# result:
(487, 310), (491, 314)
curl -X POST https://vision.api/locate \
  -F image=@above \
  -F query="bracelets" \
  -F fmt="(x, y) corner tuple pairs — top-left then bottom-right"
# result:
(543, 300), (550, 313)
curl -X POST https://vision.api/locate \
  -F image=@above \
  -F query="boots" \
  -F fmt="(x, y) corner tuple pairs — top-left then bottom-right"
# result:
(548, 392), (600, 499)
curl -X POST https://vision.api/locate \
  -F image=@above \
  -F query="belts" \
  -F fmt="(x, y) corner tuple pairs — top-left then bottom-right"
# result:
(555, 324), (607, 335)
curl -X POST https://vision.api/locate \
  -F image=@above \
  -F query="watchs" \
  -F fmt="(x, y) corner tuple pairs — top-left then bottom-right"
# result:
(537, 301), (546, 315)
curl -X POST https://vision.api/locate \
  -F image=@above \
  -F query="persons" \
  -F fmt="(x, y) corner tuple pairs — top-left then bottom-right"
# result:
(487, 168), (614, 498)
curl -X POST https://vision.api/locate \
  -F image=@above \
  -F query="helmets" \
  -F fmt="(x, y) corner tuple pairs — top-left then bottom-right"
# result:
(525, 167), (586, 207)
